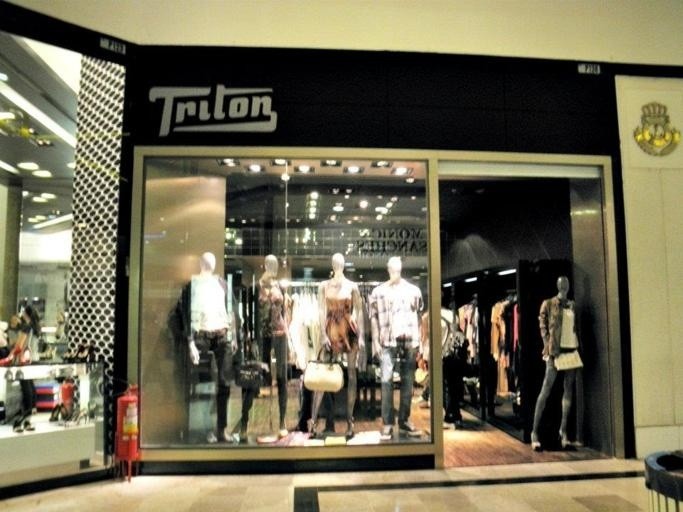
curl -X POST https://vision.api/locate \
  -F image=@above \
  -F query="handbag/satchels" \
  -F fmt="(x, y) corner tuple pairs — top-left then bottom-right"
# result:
(553, 349), (584, 372)
(304, 346), (344, 392)
(235, 369), (260, 387)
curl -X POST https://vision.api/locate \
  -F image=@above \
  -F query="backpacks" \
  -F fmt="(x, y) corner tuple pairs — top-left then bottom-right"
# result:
(441, 310), (467, 363)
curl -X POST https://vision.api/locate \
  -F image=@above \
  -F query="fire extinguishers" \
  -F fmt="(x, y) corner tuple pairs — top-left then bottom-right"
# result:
(61, 378), (76, 414)
(113, 379), (139, 461)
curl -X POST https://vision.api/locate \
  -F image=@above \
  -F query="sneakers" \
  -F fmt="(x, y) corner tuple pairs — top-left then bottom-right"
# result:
(379, 425), (394, 440)
(399, 423), (422, 436)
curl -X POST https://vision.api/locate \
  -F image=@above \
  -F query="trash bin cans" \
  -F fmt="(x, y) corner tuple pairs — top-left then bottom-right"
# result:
(644, 450), (683, 512)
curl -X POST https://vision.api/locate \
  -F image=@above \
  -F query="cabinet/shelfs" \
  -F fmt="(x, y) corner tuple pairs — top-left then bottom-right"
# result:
(0, 362), (95, 473)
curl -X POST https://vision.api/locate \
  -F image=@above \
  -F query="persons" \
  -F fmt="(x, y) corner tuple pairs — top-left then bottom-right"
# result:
(367, 255), (423, 434)
(232, 254), (297, 441)
(7, 303), (41, 417)
(303, 251), (360, 448)
(440, 289), (464, 429)
(529, 273), (586, 452)
(177, 251), (236, 444)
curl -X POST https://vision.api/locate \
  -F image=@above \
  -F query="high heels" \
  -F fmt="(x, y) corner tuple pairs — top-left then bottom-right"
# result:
(0, 340), (31, 366)
(48, 404), (88, 426)
(62, 345), (94, 361)
(13, 419), (35, 433)
